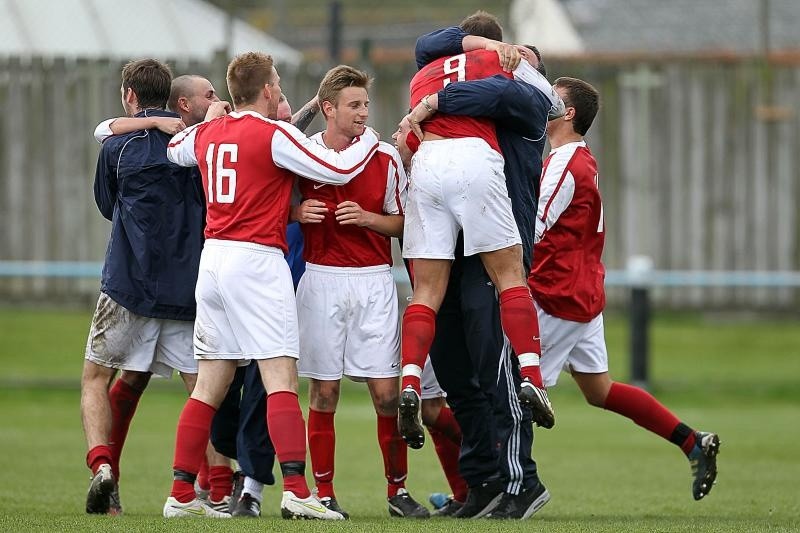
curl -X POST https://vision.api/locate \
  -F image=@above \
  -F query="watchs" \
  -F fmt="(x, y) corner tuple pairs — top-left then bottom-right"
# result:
(421, 94), (434, 111)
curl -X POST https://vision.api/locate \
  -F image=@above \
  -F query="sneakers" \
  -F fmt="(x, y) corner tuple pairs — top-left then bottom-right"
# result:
(385, 491), (429, 519)
(319, 497), (349, 520)
(450, 481), (507, 519)
(397, 388), (425, 450)
(430, 499), (466, 518)
(205, 494), (232, 513)
(110, 477), (123, 513)
(280, 490), (346, 521)
(228, 470), (247, 513)
(231, 492), (262, 518)
(488, 481), (551, 520)
(194, 481), (211, 505)
(518, 380), (555, 429)
(163, 497), (232, 520)
(688, 431), (721, 501)
(85, 464), (114, 515)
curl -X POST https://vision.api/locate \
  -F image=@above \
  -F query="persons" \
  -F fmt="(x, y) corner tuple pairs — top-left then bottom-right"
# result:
(78, 56), (234, 520)
(207, 91), (308, 519)
(526, 75), (722, 499)
(289, 63), (432, 518)
(92, 72), (223, 514)
(408, 23), (552, 522)
(396, 10), (568, 452)
(164, 51), (379, 524)
(388, 106), (472, 518)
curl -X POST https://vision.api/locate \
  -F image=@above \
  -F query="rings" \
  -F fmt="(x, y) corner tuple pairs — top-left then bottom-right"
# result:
(411, 117), (418, 124)
